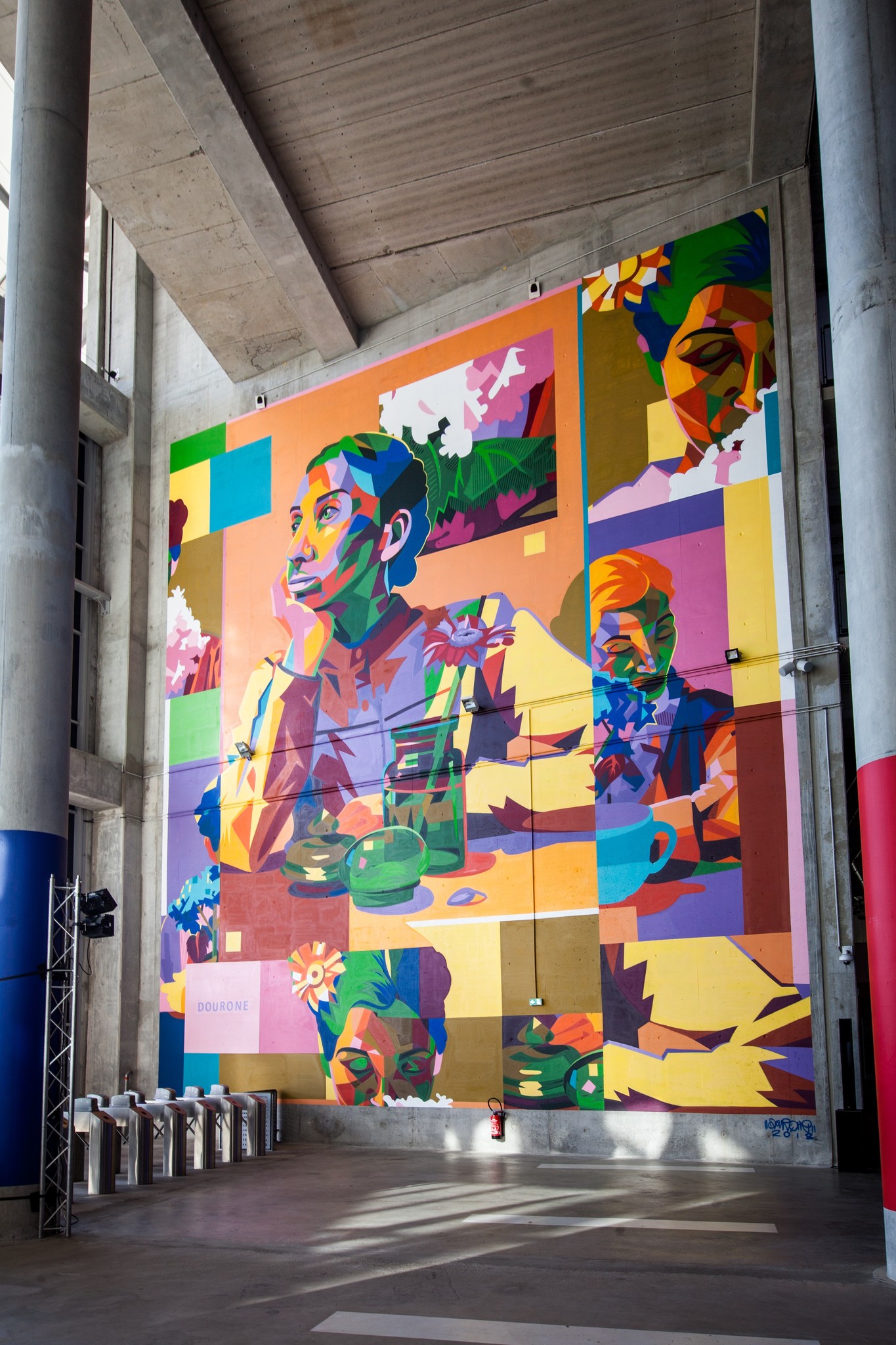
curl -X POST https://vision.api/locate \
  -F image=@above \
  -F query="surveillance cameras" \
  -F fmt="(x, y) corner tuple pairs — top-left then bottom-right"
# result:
(838, 954), (853, 965)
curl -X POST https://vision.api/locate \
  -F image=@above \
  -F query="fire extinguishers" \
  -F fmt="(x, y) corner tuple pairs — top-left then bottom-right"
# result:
(488, 1098), (506, 1139)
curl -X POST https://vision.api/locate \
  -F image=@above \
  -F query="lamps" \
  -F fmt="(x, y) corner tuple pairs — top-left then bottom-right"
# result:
(460, 696), (479, 712)
(79, 888), (118, 939)
(234, 741), (255, 761)
(725, 648), (742, 664)
(778, 662), (795, 677)
(796, 660), (813, 675)
